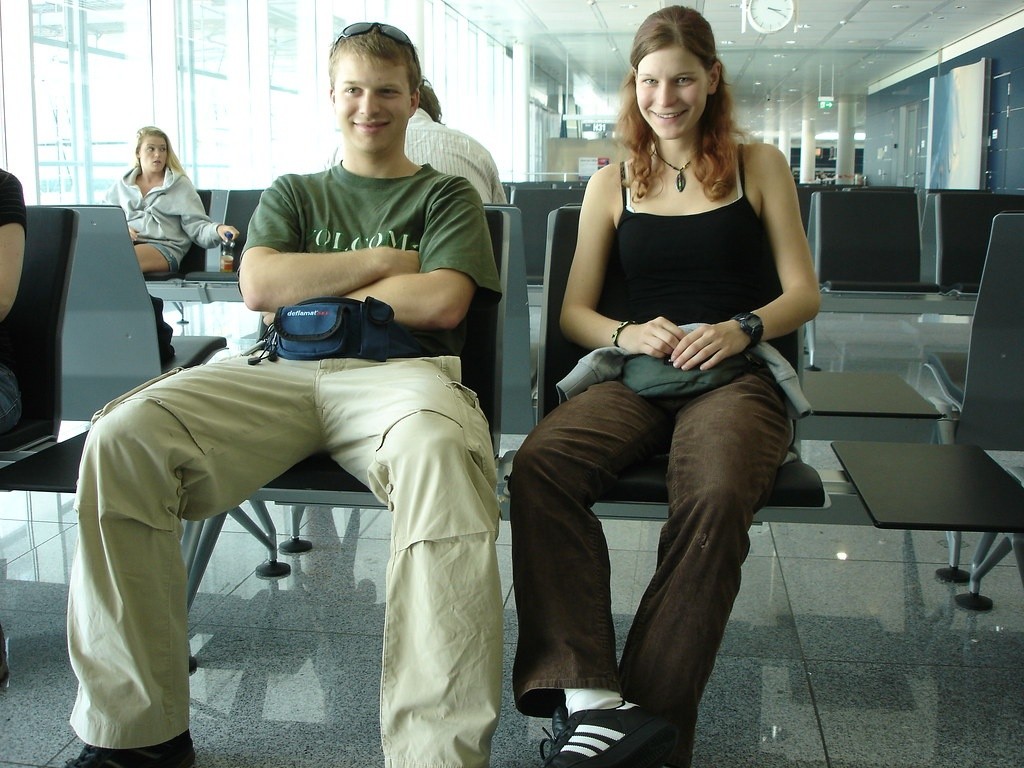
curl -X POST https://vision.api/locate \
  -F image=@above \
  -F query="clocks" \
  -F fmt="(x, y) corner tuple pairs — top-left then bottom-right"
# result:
(747, 0), (793, 33)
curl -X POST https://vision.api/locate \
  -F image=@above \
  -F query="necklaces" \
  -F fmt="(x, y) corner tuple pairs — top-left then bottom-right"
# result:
(654, 143), (692, 193)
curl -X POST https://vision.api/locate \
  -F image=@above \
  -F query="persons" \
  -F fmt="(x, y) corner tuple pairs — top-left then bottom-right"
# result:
(0, 168), (27, 694)
(322, 74), (508, 207)
(508, 5), (822, 768)
(101, 126), (240, 274)
(65, 21), (504, 768)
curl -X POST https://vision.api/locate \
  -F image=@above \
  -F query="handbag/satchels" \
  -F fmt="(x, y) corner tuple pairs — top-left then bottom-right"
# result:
(621, 353), (761, 412)
(274, 296), (445, 361)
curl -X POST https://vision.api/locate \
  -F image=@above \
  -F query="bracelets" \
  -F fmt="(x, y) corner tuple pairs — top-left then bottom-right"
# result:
(612, 319), (639, 348)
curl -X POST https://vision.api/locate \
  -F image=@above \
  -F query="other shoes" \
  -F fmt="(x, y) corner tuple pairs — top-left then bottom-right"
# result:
(63, 729), (195, 768)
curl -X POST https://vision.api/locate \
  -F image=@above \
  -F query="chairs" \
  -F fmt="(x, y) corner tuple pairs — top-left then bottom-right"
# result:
(0, 180), (1024, 611)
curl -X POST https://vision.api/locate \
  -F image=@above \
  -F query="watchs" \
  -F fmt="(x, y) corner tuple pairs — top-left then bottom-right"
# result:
(732, 312), (764, 355)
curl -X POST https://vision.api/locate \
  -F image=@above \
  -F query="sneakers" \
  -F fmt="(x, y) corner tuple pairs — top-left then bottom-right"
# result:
(538, 705), (678, 768)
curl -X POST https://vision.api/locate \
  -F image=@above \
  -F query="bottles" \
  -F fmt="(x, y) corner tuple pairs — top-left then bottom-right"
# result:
(220, 232), (236, 274)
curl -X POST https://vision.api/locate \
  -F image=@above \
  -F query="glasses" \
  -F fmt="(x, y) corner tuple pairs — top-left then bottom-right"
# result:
(334, 22), (416, 63)
